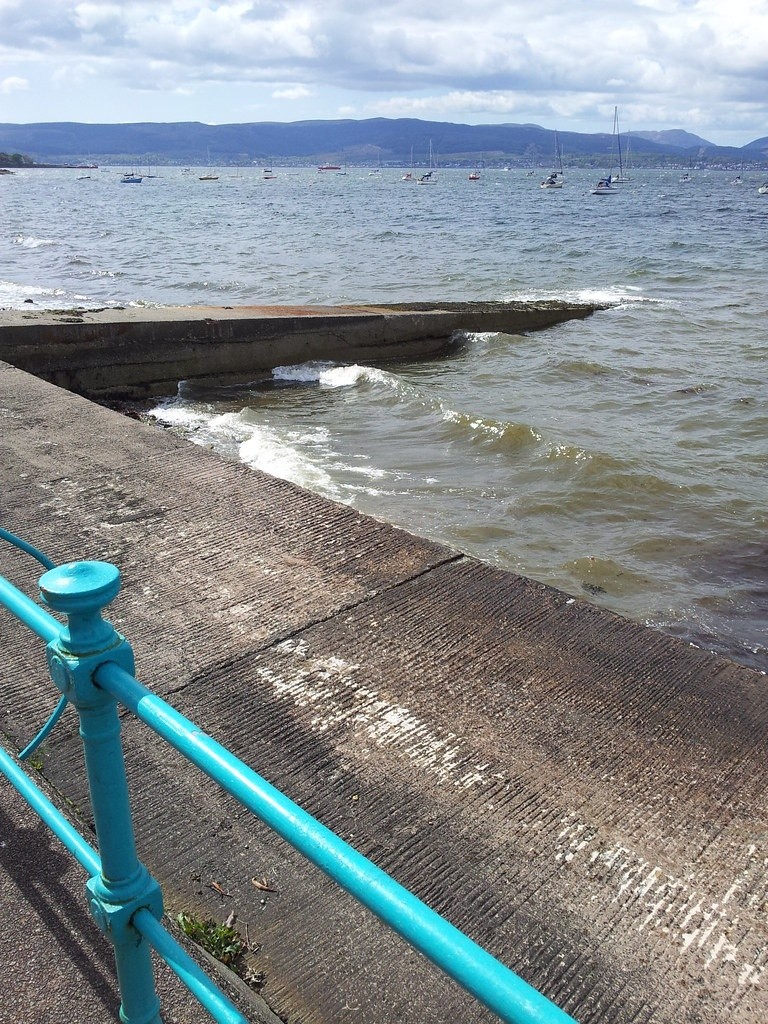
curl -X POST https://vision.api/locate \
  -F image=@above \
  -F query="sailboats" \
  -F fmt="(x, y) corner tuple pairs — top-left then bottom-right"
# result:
(402, 138), (439, 185)
(120, 156), (162, 185)
(540, 129), (565, 189)
(589, 106), (635, 194)
(679, 155), (695, 183)
(197, 145), (218, 179)
(730, 154), (744, 186)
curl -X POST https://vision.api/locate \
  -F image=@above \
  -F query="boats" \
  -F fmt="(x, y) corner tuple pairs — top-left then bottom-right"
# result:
(317, 165), (347, 176)
(757, 182), (768, 194)
(0, 168), (15, 176)
(368, 169), (383, 176)
(470, 171), (480, 180)
